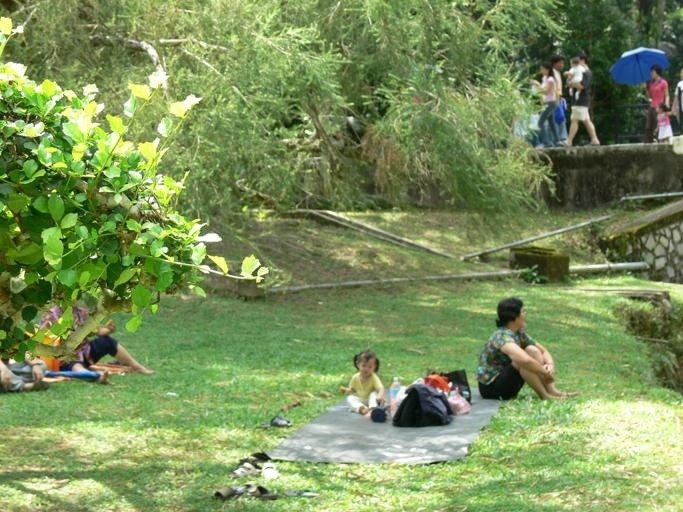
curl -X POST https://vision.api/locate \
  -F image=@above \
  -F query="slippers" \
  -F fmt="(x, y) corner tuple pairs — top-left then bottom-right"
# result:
(556, 141), (574, 147)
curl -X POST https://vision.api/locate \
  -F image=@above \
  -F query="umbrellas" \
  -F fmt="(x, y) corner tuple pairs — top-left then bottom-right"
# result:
(608, 46), (670, 101)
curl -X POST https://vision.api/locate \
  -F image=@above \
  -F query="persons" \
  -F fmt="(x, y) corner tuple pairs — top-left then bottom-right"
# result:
(0, 350), (48, 392)
(341, 350), (385, 415)
(477, 298), (579, 401)
(646, 64), (671, 142)
(671, 66), (683, 135)
(510, 51), (601, 147)
(40, 281), (155, 383)
(652, 103), (673, 142)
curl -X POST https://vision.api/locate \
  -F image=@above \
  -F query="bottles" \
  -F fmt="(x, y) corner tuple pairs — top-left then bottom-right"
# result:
(389, 376), (402, 417)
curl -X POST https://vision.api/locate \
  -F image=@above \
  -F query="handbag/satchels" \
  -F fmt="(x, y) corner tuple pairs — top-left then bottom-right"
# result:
(391, 383), (454, 428)
(425, 369), (473, 417)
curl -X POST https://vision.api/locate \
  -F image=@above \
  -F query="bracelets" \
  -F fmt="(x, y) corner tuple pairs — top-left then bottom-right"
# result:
(31, 363), (40, 367)
